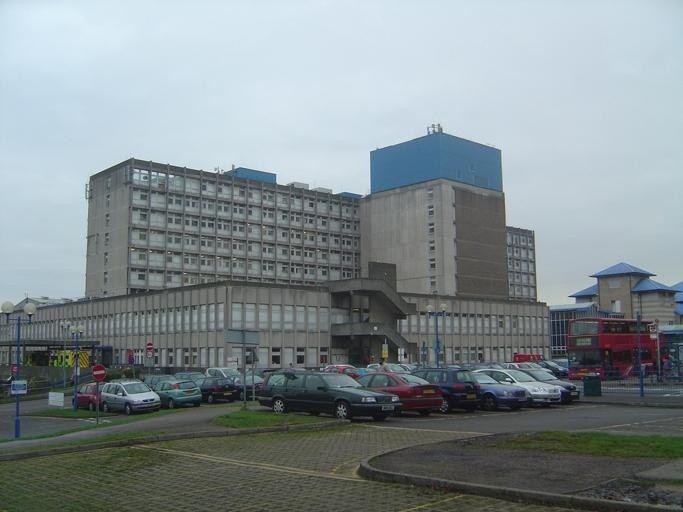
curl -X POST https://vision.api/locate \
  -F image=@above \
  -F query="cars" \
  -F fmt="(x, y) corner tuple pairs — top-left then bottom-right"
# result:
(145, 374), (176, 390)
(71, 381), (110, 411)
(150, 380), (203, 410)
(175, 372), (205, 383)
(230, 376), (265, 402)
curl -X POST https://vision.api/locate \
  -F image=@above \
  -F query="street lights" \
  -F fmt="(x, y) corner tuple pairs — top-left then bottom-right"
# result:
(59, 321), (71, 388)
(1, 300), (36, 439)
(424, 302), (448, 368)
(70, 325), (86, 411)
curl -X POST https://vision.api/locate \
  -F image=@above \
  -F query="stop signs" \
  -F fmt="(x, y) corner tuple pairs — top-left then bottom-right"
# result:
(147, 343), (153, 350)
(92, 365), (107, 381)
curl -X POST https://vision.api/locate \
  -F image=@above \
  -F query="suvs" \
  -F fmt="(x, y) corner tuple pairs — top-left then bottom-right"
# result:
(194, 376), (235, 404)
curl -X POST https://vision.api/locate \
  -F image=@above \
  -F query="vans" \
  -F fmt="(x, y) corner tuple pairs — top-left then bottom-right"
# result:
(205, 367), (242, 381)
(101, 378), (162, 415)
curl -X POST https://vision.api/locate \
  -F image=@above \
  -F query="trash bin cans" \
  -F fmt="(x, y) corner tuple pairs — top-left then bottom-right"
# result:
(583, 372), (602, 397)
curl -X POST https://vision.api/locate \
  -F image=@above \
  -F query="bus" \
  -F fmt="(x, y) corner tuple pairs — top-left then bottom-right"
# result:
(566, 316), (669, 381)
